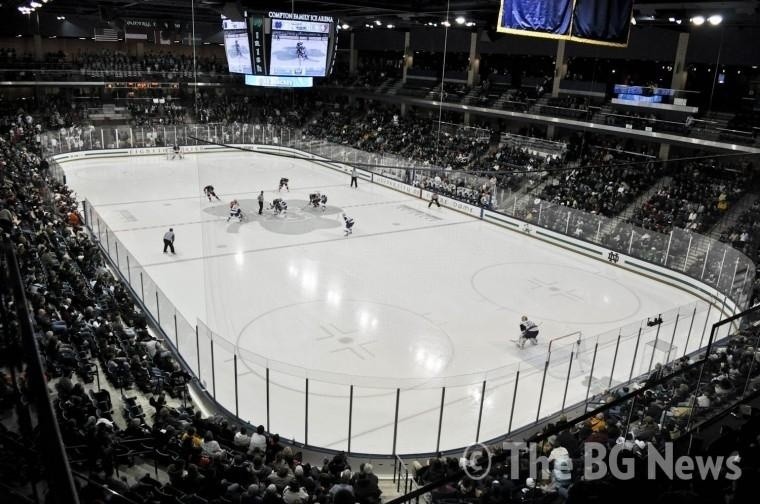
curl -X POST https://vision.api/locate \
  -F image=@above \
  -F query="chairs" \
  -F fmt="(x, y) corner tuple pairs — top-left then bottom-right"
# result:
(0, 50), (760, 504)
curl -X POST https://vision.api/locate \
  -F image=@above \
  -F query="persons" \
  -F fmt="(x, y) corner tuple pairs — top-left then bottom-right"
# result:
(295, 42), (307, 59)
(234, 40), (243, 57)
(0, 47), (760, 504)
